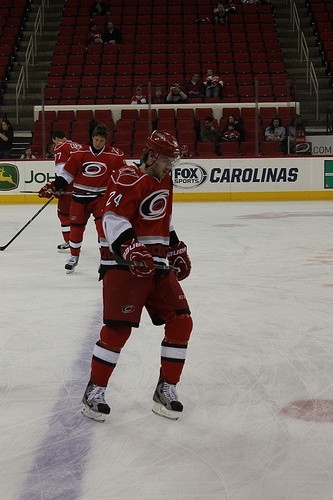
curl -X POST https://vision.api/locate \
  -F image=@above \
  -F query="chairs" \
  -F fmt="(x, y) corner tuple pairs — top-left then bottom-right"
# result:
(306, 0), (333, 89)
(0, 0), (299, 160)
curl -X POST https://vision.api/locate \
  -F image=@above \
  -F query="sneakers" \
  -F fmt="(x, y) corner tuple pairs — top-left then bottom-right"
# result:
(57, 243), (71, 252)
(81, 372), (111, 422)
(64, 254), (79, 274)
(152, 366), (184, 420)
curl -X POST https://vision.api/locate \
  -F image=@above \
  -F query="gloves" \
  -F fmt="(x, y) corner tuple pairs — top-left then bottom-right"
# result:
(120, 238), (156, 278)
(167, 241), (191, 282)
(38, 181), (61, 198)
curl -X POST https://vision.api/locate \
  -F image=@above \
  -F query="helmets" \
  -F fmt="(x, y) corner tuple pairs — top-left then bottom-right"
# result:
(145, 129), (180, 160)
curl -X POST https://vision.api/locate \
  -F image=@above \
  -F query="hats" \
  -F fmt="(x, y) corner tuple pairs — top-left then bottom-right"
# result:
(136, 87), (142, 91)
(174, 83), (179, 87)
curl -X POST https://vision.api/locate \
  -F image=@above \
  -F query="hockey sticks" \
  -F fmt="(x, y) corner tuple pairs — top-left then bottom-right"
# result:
(20, 191), (106, 196)
(0, 195), (55, 251)
(100, 259), (180, 272)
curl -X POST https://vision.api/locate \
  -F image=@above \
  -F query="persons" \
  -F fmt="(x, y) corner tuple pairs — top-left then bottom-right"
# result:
(81, 130), (193, 422)
(0, 0), (303, 252)
(37, 124), (127, 274)
